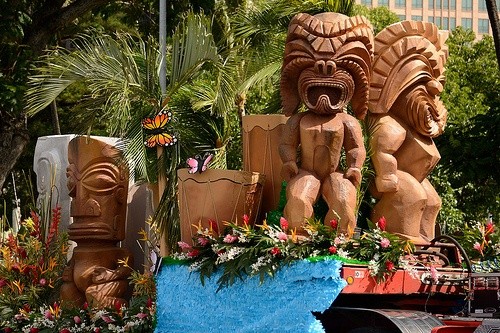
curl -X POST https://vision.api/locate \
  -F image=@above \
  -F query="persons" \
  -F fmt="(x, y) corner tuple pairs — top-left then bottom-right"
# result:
(277, 10), (375, 237)
(59, 135), (135, 315)
(364, 19), (451, 244)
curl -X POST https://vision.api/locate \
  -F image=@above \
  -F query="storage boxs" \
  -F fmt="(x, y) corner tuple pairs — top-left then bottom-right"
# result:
(403, 270), (470, 295)
(341, 266), (403, 294)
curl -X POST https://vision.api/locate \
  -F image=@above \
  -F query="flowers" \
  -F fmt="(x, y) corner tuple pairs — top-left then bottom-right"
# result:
(454, 222), (500, 271)
(172, 217), (412, 292)
(0, 163), (155, 333)
